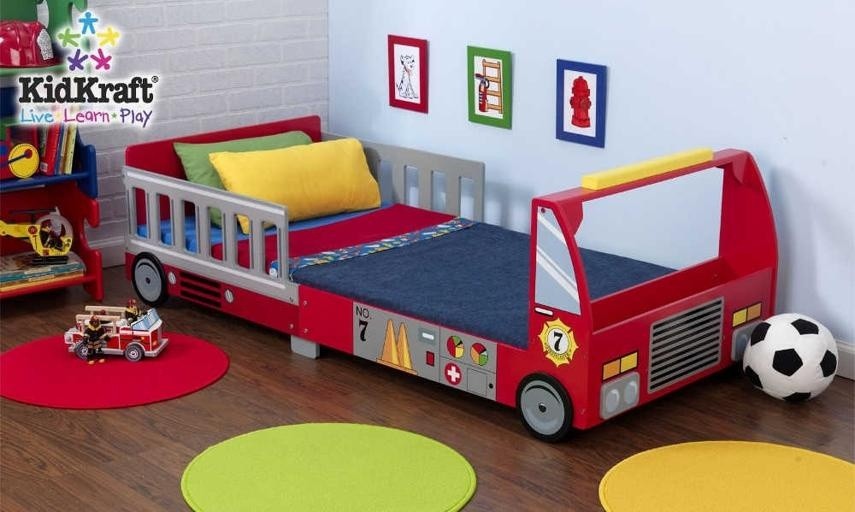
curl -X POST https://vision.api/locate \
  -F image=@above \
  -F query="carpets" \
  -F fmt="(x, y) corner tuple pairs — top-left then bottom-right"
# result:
(597, 435), (855, 512)
(180, 423), (478, 511)
(0, 329), (230, 413)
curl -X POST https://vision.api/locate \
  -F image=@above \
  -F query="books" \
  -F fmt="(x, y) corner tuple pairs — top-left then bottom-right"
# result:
(0, 253), (86, 291)
(40, 118), (77, 176)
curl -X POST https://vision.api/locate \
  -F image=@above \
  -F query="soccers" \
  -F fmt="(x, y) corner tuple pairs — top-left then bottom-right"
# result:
(743, 312), (838, 404)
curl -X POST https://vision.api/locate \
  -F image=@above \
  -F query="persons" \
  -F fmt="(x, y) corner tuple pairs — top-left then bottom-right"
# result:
(63, 297), (169, 366)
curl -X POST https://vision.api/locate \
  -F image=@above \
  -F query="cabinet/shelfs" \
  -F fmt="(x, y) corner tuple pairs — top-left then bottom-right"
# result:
(0, 0), (104, 309)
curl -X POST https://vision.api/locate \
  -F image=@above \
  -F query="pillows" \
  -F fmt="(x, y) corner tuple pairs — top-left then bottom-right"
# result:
(206, 135), (382, 238)
(170, 128), (315, 232)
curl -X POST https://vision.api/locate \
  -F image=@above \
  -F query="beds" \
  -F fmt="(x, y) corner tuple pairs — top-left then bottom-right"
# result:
(116, 111), (781, 446)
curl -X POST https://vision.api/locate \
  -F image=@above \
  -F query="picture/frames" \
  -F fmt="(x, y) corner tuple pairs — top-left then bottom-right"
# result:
(465, 44), (514, 130)
(555, 57), (607, 149)
(386, 34), (429, 114)
